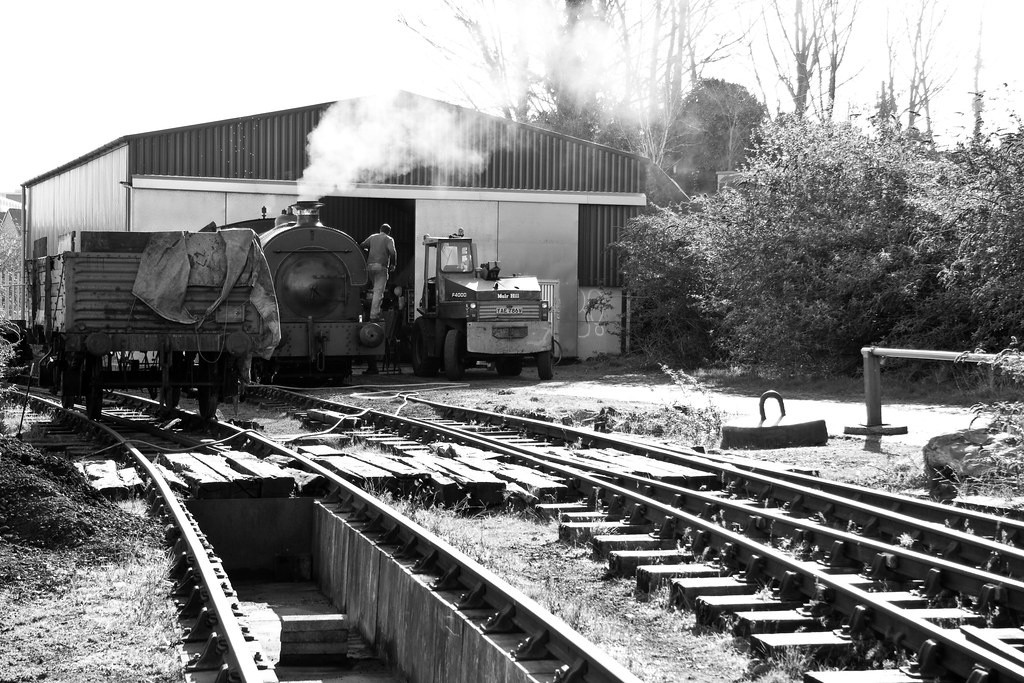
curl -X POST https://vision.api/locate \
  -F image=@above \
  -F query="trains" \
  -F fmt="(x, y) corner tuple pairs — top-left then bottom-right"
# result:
(216, 201), (386, 386)
(23, 228), (274, 422)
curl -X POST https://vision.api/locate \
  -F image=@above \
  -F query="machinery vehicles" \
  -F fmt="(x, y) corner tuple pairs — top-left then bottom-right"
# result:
(410, 228), (555, 379)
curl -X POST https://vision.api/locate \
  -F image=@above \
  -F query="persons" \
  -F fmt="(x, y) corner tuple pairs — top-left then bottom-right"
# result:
(360, 222), (397, 323)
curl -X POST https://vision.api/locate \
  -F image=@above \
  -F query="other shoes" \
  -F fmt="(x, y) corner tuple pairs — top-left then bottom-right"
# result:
(370, 317), (380, 323)
